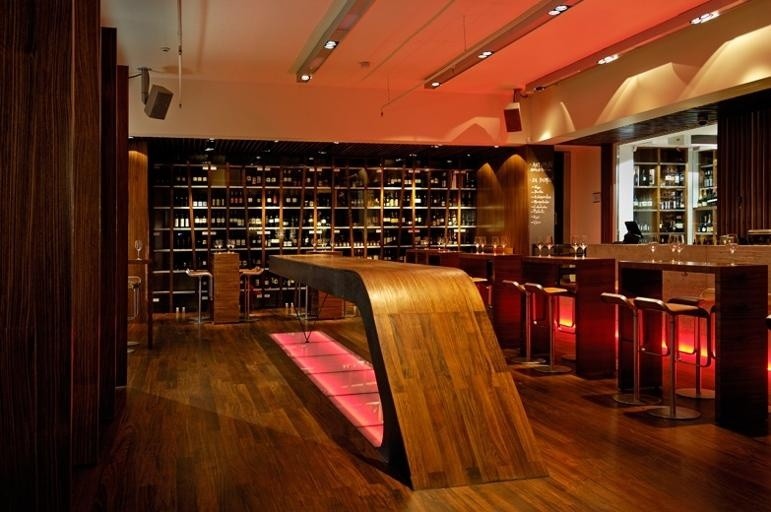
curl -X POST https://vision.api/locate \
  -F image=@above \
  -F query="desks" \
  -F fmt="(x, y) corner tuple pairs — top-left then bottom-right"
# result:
(127, 259), (152, 350)
(210, 252), (239, 324)
(617, 259), (768, 426)
(405, 249), (448, 266)
(307, 251), (345, 320)
(440, 252), (522, 348)
(521, 255), (617, 380)
(269, 252), (552, 491)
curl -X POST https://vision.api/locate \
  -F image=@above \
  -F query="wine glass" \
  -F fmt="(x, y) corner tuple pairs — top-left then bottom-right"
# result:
(536, 239), (545, 257)
(571, 239), (591, 259)
(667, 232), (687, 265)
(474, 234), (510, 256)
(546, 239), (554, 258)
(721, 233), (740, 266)
(648, 235), (660, 264)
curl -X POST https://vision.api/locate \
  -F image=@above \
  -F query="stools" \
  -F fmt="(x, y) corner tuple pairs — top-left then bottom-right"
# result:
(184, 269), (213, 325)
(671, 286), (719, 402)
(287, 281), (306, 317)
(603, 290), (664, 409)
(239, 265), (266, 323)
(346, 303), (361, 318)
(633, 295), (707, 423)
(475, 278), (574, 378)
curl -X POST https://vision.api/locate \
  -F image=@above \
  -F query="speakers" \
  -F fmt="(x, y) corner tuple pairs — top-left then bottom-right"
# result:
(143, 84), (174, 120)
(503, 102), (523, 132)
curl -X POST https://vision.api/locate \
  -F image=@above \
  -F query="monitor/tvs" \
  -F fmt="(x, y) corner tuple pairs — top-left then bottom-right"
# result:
(625, 221), (641, 235)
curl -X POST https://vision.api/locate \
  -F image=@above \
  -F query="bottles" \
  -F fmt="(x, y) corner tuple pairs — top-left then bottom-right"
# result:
(181, 307), (187, 323)
(284, 302), (290, 316)
(335, 239), (381, 246)
(175, 306), (180, 321)
(693, 168), (715, 245)
(255, 276), (301, 289)
(243, 252), (261, 268)
(560, 262), (577, 283)
(396, 153), (423, 168)
(384, 231), (398, 245)
(172, 170), (331, 253)
(431, 230), (459, 245)
(197, 255), (203, 270)
(352, 170), (477, 227)
(291, 302), (295, 316)
(635, 164), (686, 244)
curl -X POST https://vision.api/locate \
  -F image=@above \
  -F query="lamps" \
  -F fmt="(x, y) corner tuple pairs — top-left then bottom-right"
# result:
(424, 1), (585, 89)
(527, 0), (749, 95)
(295, 1), (378, 83)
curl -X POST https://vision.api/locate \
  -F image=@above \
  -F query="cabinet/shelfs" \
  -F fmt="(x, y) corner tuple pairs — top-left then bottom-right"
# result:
(692, 147), (719, 246)
(150, 163), (475, 313)
(634, 147), (688, 245)
